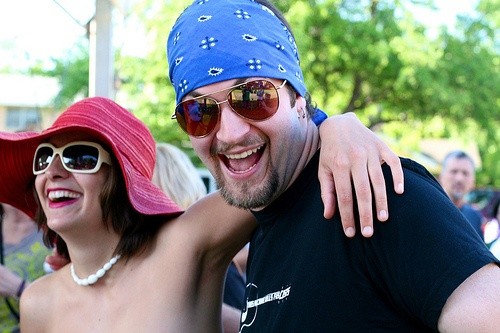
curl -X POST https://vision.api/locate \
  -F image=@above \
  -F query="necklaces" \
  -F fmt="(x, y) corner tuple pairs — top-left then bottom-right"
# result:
(71, 247), (124, 286)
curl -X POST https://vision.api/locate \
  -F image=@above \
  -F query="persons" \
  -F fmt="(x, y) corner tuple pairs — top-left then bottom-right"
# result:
(0, 0), (500, 333)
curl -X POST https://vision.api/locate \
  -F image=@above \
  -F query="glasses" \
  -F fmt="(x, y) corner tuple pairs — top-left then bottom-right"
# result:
(171, 79), (287, 138)
(33, 141), (113, 175)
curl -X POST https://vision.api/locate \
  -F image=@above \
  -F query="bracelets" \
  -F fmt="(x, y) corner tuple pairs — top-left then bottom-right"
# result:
(16, 279), (25, 297)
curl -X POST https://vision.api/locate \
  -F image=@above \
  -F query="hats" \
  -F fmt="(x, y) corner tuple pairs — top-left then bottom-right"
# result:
(0, 97), (186, 242)
